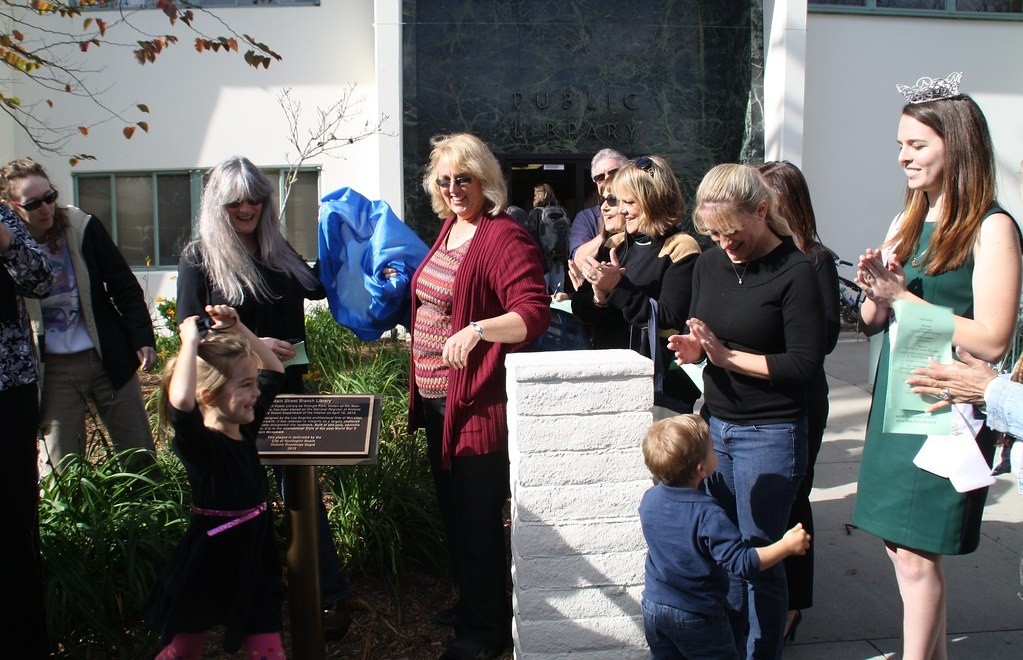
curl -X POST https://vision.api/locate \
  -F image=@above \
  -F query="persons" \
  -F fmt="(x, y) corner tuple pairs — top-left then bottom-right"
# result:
(1, 157), (340, 660)
(853, 71), (1023, 660)
(508, 146), (1023, 660)
(383, 133), (551, 660)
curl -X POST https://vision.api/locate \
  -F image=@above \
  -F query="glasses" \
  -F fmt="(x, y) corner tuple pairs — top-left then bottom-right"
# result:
(593, 167), (620, 183)
(9, 187), (58, 211)
(631, 156), (654, 179)
(597, 193), (618, 208)
(227, 196), (263, 208)
(197, 315), (237, 335)
(435, 174), (475, 188)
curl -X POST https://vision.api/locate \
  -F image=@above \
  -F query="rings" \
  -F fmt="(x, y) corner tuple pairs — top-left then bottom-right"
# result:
(599, 266), (608, 271)
(586, 272), (592, 277)
(573, 272), (578, 277)
(940, 389), (951, 400)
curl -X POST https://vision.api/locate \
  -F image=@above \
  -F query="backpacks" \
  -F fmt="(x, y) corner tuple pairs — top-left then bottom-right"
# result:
(532, 206), (571, 260)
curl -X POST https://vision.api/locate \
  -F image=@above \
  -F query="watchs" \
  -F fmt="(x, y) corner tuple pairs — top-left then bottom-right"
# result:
(470, 321), (483, 342)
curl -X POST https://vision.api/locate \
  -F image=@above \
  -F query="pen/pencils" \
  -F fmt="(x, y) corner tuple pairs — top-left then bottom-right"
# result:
(553, 282), (561, 298)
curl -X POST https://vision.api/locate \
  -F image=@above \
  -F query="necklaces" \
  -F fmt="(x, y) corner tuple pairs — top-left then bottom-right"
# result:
(730, 249), (754, 285)
(911, 242), (930, 267)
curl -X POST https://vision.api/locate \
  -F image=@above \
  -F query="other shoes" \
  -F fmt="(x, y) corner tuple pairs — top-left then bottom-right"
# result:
(431, 607), (456, 627)
(438, 635), (497, 660)
(321, 600), (352, 643)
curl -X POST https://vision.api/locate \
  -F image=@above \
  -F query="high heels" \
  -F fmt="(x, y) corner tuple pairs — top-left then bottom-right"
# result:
(783, 609), (802, 645)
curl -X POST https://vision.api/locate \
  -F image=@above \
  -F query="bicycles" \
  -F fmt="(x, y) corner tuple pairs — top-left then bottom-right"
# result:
(834, 256), (867, 334)
